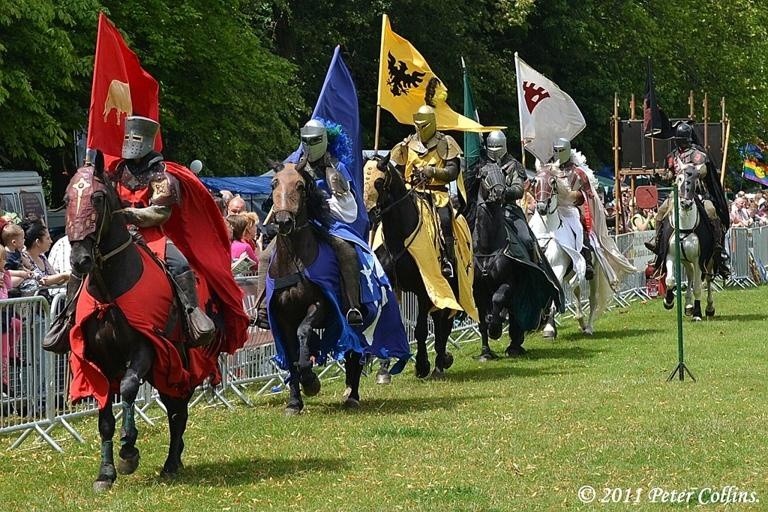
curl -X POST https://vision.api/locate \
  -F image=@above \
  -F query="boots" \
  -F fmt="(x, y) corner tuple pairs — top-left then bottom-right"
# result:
(247, 293), (270, 330)
(174, 271), (213, 343)
(347, 311), (362, 324)
(67, 274), (81, 323)
(644, 221), (662, 254)
(583, 240), (593, 280)
(443, 236), (454, 275)
(712, 219), (723, 252)
(530, 247), (538, 265)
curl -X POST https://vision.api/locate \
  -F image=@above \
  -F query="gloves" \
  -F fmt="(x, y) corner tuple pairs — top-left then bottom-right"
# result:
(422, 166), (447, 181)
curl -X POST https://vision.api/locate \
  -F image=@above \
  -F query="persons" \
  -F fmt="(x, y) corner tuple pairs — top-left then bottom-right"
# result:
(649, 120), (726, 252)
(1, 180), (768, 393)
(476, 129), (544, 268)
(110, 116), (219, 344)
(387, 103), (465, 279)
(297, 116), (369, 327)
(546, 136), (597, 282)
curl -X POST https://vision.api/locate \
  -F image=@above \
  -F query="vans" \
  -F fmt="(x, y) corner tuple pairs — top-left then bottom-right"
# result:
(0, 170), (50, 254)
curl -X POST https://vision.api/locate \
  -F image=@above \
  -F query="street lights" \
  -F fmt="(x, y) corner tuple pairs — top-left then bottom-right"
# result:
(189, 159), (203, 177)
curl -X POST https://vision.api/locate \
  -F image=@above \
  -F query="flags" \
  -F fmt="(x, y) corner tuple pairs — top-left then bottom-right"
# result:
(458, 54), (482, 174)
(511, 48), (588, 165)
(302, 48), (365, 201)
(641, 57), (679, 142)
(85, 9), (166, 162)
(740, 156), (768, 186)
(374, 12), (511, 133)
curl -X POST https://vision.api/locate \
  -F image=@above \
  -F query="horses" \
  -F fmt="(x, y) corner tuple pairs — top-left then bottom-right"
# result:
(362, 150), (454, 385)
(528, 157), (612, 337)
(472, 162), (552, 362)
(61, 149), (227, 493)
(658, 157), (715, 321)
(268, 153), (366, 416)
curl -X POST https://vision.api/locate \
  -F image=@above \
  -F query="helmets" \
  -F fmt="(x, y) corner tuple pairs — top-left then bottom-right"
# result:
(486, 130), (508, 161)
(300, 120), (328, 163)
(414, 105), (436, 142)
(553, 138), (571, 164)
(675, 123), (693, 142)
(122, 116), (160, 160)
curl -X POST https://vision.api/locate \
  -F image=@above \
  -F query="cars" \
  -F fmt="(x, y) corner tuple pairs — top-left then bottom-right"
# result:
(43, 210), (66, 256)
